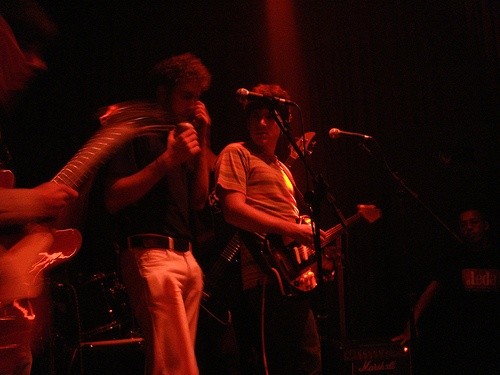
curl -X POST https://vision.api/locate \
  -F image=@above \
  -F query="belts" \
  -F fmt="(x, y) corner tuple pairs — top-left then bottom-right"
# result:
(119, 236), (190, 253)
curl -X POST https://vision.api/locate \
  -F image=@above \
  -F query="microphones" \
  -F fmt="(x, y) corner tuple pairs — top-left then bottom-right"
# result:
(328, 128), (374, 142)
(235, 88), (296, 107)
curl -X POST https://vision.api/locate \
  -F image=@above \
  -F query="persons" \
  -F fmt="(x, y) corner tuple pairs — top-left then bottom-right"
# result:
(0, 13), (80, 229)
(215, 83), (326, 375)
(390, 208), (500, 346)
(103, 52), (212, 374)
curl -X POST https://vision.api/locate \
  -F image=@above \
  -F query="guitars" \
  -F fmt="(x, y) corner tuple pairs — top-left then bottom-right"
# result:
(0, 103), (176, 322)
(263, 204), (382, 294)
(196, 129), (317, 326)
